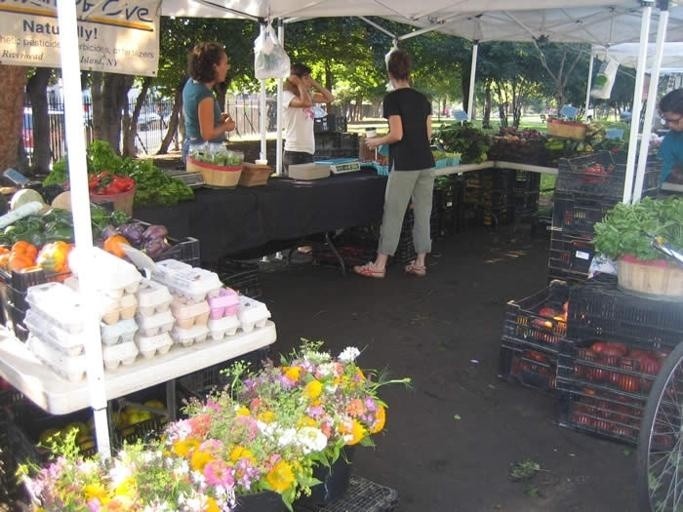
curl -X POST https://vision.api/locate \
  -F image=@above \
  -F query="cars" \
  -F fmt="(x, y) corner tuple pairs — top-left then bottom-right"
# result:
(22, 105), (173, 149)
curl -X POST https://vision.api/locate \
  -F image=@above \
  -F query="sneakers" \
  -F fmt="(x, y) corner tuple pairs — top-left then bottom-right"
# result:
(405, 260), (426, 275)
(354, 261), (386, 277)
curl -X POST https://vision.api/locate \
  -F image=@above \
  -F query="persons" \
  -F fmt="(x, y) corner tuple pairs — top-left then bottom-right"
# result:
(351, 49), (440, 282)
(281, 62), (335, 169)
(654, 88), (682, 192)
(180, 43), (234, 165)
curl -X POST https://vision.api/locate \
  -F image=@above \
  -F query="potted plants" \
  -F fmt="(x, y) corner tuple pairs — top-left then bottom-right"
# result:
(590, 195), (682, 305)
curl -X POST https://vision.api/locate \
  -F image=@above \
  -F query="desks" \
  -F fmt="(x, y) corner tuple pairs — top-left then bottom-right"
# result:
(496, 159), (559, 227)
(0, 320), (277, 457)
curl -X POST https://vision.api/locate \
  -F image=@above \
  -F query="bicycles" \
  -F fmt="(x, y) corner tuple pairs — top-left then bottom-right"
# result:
(637, 230), (682, 512)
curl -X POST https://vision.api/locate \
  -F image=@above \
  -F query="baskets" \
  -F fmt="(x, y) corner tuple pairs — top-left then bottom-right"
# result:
(312, 235), (390, 271)
(0, 217), (200, 344)
(176, 348), (283, 406)
(495, 339), (559, 398)
(553, 149), (664, 199)
(566, 283), (682, 353)
(213, 256), (262, 299)
(447, 158), (460, 166)
(389, 168), (540, 271)
(313, 113), (347, 134)
(312, 132), (360, 161)
(547, 379), (682, 451)
(0, 388), (188, 505)
(555, 335), (683, 406)
(375, 165), (391, 177)
(486, 128), (610, 168)
(549, 191), (622, 241)
(434, 157), (449, 168)
(501, 279), (568, 354)
(547, 239), (617, 284)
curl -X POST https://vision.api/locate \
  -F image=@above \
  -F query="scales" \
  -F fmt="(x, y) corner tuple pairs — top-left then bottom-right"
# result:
(314, 158), (361, 175)
(164, 169), (205, 191)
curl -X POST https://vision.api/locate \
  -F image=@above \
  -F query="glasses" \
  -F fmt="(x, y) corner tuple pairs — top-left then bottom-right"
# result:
(660, 116), (682, 125)
